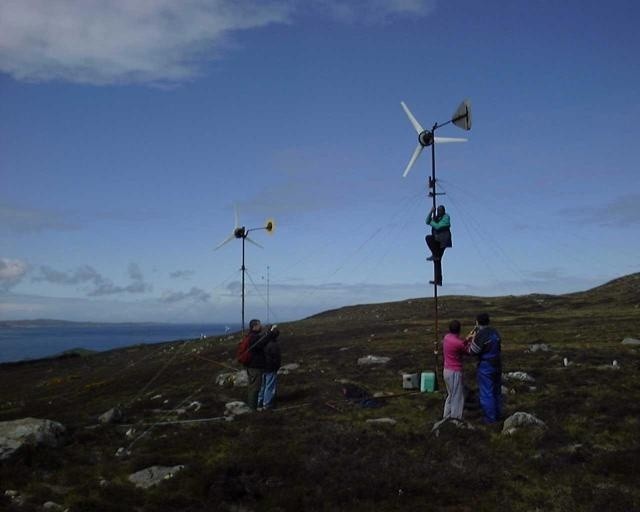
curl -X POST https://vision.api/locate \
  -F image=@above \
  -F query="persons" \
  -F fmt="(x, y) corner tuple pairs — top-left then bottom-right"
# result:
(256, 329), (281, 412)
(425, 205), (452, 287)
(442, 320), (477, 421)
(468, 312), (504, 423)
(247, 319), (277, 407)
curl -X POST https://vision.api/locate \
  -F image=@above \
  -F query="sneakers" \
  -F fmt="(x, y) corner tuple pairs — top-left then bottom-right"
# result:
(430, 281), (442, 286)
(426, 256), (439, 260)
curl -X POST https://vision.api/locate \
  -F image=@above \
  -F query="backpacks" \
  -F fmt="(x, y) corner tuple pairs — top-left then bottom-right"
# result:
(237, 334), (252, 362)
